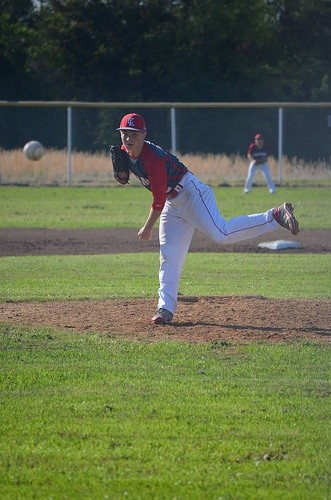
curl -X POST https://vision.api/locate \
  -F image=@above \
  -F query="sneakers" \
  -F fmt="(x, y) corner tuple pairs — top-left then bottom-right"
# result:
(152, 308), (172, 324)
(273, 202), (298, 234)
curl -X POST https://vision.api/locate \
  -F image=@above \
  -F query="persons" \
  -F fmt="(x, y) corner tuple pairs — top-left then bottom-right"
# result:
(109, 112), (301, 326)
(242, 132), (279, 197)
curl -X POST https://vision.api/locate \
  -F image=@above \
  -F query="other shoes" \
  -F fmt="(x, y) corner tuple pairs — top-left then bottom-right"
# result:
(244, 189), (250, 193)
(269, 190), (275, 193)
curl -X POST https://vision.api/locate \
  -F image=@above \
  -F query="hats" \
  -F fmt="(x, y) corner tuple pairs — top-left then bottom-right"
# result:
(116, 113), (146, 131)
(255, 134), (262, 139)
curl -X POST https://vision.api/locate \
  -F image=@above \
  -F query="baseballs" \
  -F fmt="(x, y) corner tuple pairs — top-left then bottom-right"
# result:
(24, 141), (45, 160)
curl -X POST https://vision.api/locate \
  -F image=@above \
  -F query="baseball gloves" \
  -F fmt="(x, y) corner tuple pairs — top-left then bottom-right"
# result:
(255, 157), (265, 164)
(109, 144), (132, 185)
